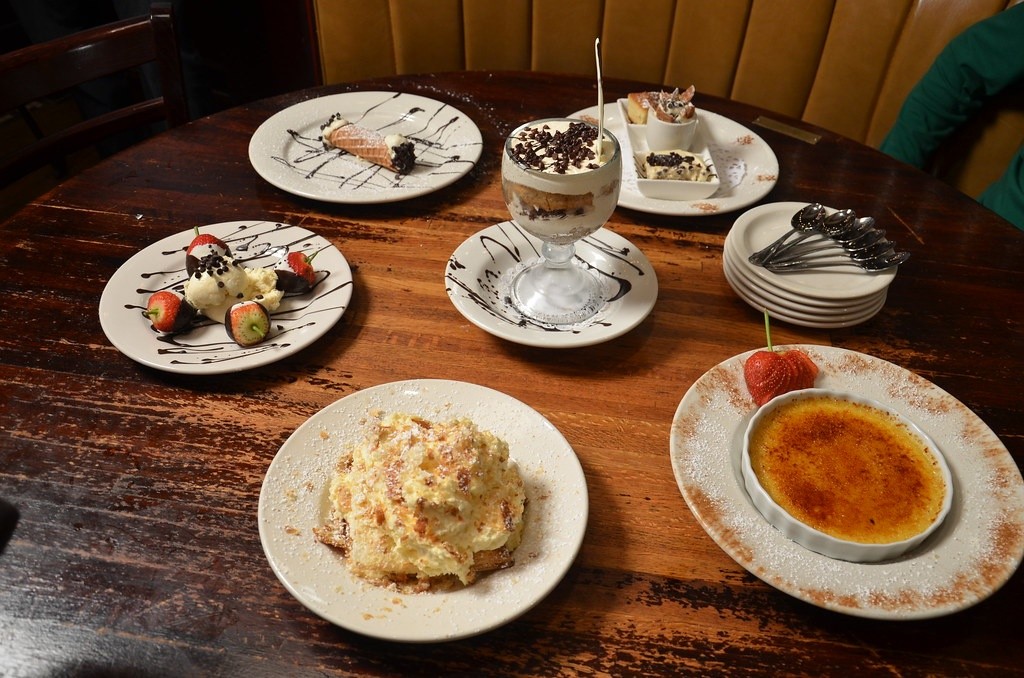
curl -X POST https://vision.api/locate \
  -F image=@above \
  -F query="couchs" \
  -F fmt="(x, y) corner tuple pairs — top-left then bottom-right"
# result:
(301, 0), (1024, 242)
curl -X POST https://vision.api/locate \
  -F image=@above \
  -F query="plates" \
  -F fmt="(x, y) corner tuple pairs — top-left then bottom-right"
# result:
(721, 201), (899, 328)
(249, 91), (484, 205)
(99, 221), (353, 375)
(739, 386), (954, 562)
(257, 379), (589, 642)
(444, 219), (658, 348)
(566, 102), (780, 216)
(670, 343), (1022, 618)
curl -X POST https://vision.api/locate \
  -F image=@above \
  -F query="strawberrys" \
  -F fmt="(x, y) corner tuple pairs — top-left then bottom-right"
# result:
(143, 290), (196, 332)
(186, 226), (234, 277)
(272, 250), (318, 294)
(224, 300), (270, 349)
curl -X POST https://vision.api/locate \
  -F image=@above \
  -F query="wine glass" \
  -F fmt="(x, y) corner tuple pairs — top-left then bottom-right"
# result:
(501, 118), (623, 326)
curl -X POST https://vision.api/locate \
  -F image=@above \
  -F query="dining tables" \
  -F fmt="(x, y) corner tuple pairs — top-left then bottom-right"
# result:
(1, 70), (1024, 678)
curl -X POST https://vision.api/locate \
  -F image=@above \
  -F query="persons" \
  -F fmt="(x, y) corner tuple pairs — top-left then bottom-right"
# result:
(875, 0), (1024, 240)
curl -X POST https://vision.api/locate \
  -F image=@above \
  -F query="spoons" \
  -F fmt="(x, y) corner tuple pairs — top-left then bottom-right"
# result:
(748, 203), (825, 267)
(764, 239), (897, 264)
(754, 208), (855, 260)
(766, 252), (910, 273)
(763, 227), (886, 267)
(769, 217), (875, 265)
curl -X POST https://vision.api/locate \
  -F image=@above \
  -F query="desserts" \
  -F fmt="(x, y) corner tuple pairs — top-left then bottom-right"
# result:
(644, 84), (697, 125)
(319, 112), (415, 173)
(501, 121), (623, 235)
(628, 92), (661, 125)
(184, 249), (285, 323)
(642, 149), (709, 181)
(314, 411), (528, 590)
